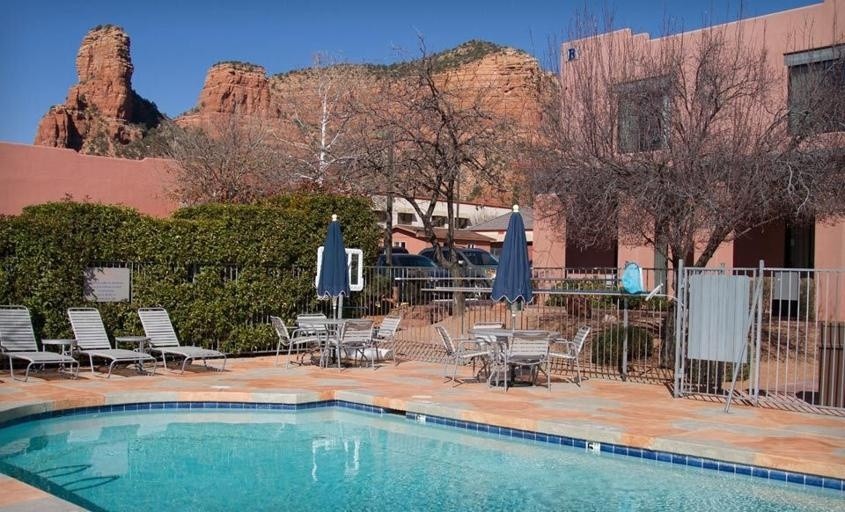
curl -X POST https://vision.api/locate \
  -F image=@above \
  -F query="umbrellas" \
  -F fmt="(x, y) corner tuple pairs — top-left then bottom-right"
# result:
(490, 203), (536, 329)
(316, 212), (352, 320)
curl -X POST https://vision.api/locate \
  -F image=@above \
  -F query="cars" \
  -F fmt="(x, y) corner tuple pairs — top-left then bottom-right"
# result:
(418, 248), (499, 298)
(366, 254), (451, 303)
